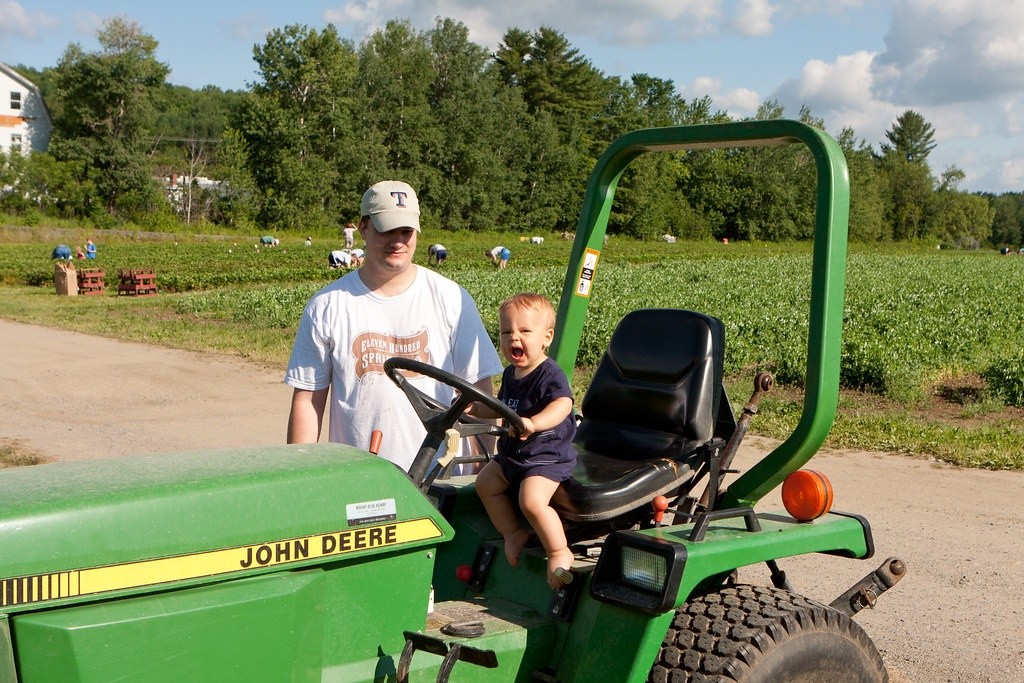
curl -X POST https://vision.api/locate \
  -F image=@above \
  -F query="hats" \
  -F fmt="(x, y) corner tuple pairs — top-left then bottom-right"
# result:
(275, 239), (279, 245)
(361, 181), (421, 233)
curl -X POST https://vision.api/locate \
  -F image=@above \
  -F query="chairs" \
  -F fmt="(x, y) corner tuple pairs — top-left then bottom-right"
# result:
(549, 309), (726, 522)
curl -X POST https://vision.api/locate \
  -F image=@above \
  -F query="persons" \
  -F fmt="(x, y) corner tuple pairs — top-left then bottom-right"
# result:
(1000, 248), (1011, 256)
(260, 236), (278, 247)
(428, 244), (446, 264)
(51, 245), (71, 259)
(84, 238), (96, 259)
(485, 246), (510, 269)
(457, 292), (578, 588)
(329, 248), (364, 270)
(305, 237), (311, 246)
(286, 179), (507, 477)
(342, 223), (358, 248)
(73, 246), (85, 260)
(531, 237), (544, 244)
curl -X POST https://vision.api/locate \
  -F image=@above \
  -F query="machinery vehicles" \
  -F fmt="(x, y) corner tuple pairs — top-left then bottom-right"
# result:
(0, 119), (906, 681)
